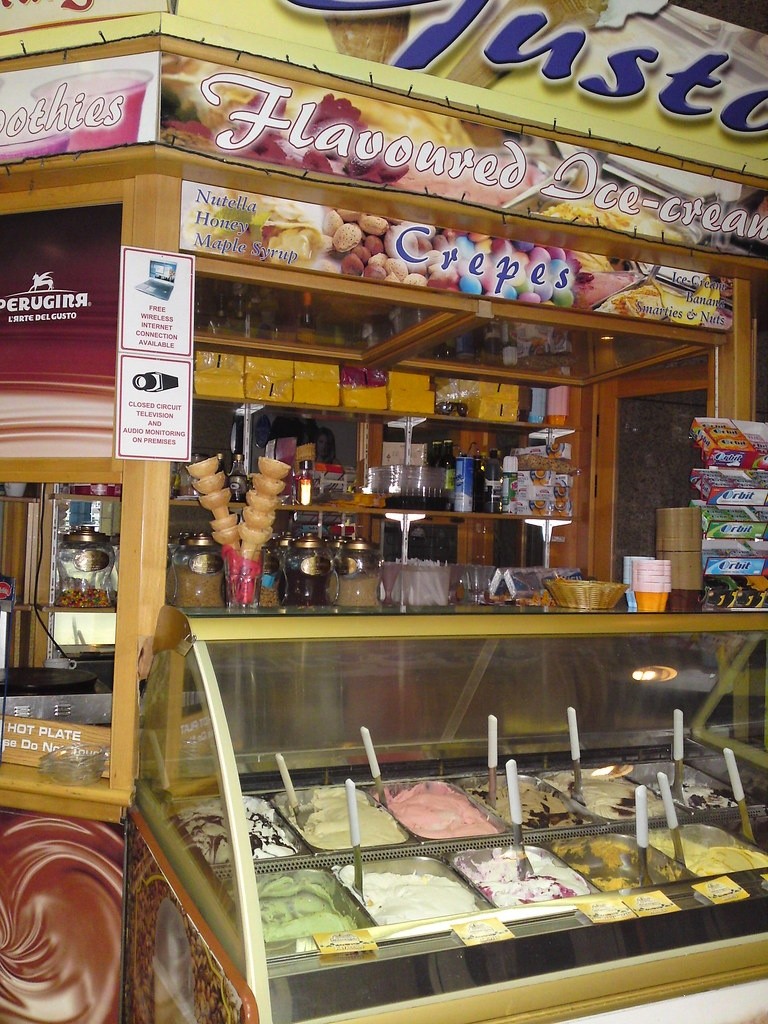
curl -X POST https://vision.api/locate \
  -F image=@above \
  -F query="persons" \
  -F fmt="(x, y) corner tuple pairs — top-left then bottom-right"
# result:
(315, 425), (340, 465)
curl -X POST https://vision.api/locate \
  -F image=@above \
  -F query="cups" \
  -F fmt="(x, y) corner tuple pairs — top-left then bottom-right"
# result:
(448, 564), (495, 602)
(43, 658), (79, 670)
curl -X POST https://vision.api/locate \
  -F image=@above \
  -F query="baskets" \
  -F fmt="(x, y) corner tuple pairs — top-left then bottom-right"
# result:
(542, 577), (630, 609)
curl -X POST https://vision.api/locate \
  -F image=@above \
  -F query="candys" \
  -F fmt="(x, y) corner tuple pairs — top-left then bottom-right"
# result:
(58, 589), (109, 607)
(326, 208), (576, 307)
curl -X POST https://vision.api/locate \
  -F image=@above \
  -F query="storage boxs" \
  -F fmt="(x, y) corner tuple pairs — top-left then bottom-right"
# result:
(517, 487), (569, 502)
(517, 501), (549, 516)
(689, 418), (768, 608)
(555, 474), (574, 488)
(549, 502), (572, 517)
(510, 443), (572, 460)
(518, 470), (555, 487)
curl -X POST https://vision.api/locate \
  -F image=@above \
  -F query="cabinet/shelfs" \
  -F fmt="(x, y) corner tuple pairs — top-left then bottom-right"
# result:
(690, 438), (768, 611)
(169, 394), (583, 521)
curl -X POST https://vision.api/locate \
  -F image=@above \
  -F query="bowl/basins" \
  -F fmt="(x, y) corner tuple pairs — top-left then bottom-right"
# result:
(368, 464), (447, 497)
(623, 556), (672, 612)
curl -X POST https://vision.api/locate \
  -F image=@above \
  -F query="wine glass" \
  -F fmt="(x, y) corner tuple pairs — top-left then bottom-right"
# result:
(379, 562), (402, 607)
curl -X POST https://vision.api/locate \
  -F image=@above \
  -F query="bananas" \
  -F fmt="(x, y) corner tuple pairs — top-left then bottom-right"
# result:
(652, 276), (721, 323)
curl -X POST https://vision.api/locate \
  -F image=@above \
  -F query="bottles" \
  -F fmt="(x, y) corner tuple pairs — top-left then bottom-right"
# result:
(484, 448), (502, 513)
(229, 454), (249, 503)
(472, 454), (484, 512)
(216, 453), (229, 488)
(297, 292), (314, 344)
(429, 442), (442, 466)
(440, 440), (456, 511)
(296, 460), (312, 506)
